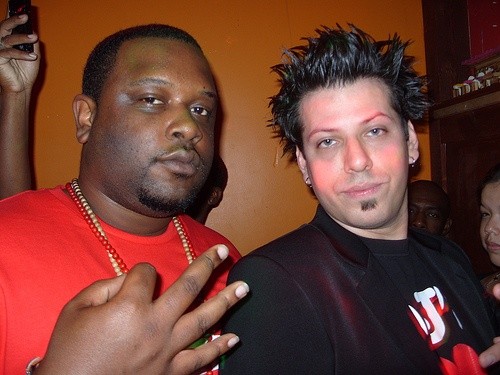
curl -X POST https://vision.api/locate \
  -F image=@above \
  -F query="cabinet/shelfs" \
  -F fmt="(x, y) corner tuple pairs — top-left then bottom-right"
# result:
(422, 0), (500, 271)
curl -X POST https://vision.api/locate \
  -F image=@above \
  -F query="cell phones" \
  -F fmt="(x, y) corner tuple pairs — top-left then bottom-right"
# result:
(8, 0), (34, 53)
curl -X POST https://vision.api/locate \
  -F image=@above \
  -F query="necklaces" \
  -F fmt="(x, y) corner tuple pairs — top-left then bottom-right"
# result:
(65, 178), (196, 276)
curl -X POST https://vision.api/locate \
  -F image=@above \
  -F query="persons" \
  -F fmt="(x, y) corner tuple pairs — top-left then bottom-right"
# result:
(223, 23), (500, 375)
(1, 23), (250, 375)
(0, 2), (228, 225)
